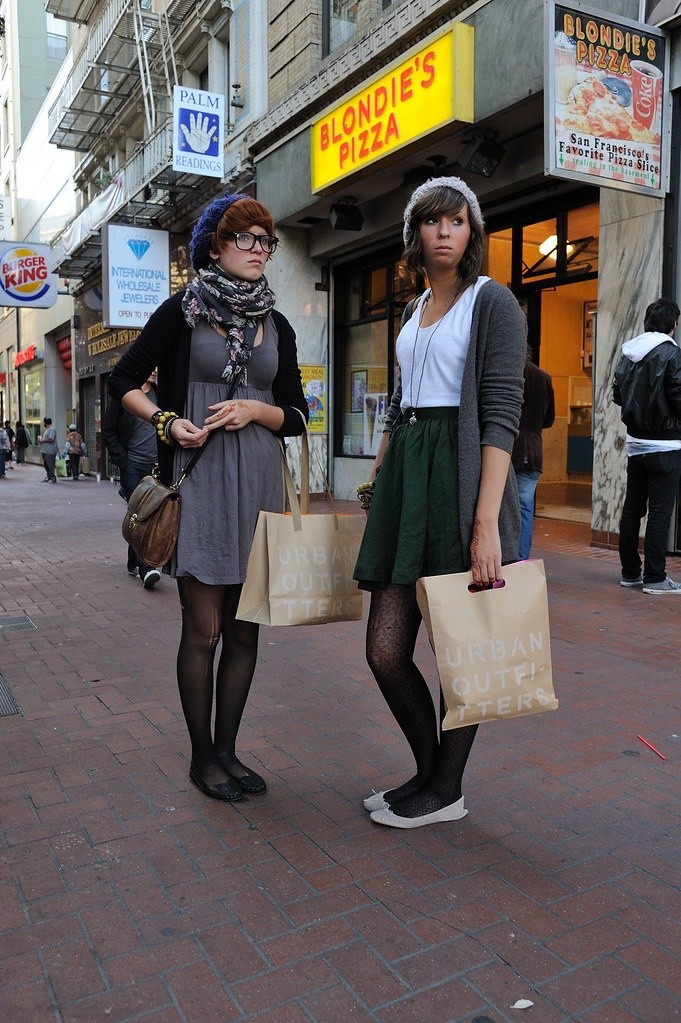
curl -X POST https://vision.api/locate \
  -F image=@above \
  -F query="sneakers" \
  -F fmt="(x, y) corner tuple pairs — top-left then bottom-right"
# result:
(621, 572), (645, 587)
(643, 577), (681, 594)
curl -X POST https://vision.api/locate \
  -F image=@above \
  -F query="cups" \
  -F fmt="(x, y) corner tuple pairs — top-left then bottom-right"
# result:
(555, 42), (577, 103)
(38, 436), (41, 441)
(630, 59), (663, 130)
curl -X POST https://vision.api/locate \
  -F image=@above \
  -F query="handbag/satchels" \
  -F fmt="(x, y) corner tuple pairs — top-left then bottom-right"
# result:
(417, 558), (559, 730)
(236, 510), (367, 626)
(122, 475), (183, 570)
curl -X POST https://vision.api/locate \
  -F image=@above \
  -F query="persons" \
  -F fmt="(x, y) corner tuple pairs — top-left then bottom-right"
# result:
(101, 366), (161, 590)
(352, 176), (530, 829)
(62, 424), (88, 481)
(611, 298), (681, 595)
(0, 420), (39, 479)
(511, 344), (556, 561)
(106, 192), (309, 802)
(37, 417), (61, 483)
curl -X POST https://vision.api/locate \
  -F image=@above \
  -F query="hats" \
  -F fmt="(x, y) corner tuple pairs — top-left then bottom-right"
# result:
(189, 193), (249, 273)
(69, 425), (77, 430)
(403, 176), (483, 251)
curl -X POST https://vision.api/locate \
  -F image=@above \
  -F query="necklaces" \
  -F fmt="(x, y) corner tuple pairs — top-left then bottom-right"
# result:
(409, 288), (459, 426)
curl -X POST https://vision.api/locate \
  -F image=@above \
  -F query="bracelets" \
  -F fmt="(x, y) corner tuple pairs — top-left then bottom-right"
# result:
(356, 482), (376, 509)
(151, 411), (180, 446)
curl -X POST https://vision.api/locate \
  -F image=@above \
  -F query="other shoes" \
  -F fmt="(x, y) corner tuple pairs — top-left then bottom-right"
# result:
(42, 476), (56, 482)
(364, 787), (468, 828)
(143, 570), (160, 589)
(73, 476), (78, 480)
(190, 758), (266, 803)
(128, 568), (140, 578)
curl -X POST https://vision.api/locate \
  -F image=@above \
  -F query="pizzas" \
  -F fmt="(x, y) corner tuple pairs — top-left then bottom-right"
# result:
(564, 76), (661, 144)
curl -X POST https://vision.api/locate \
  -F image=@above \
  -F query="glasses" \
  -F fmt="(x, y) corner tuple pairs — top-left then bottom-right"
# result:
(224, 231), (279, 254)
